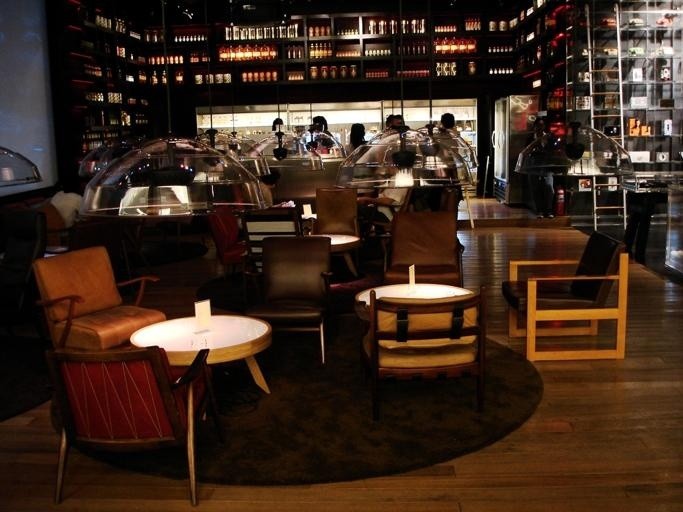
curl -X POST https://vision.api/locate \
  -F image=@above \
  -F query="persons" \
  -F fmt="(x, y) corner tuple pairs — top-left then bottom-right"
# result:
(355, 148), (416, 234)
(382, 112), (405, 130)
(43, 173), (83, 239)
(618, 183), (656, 267)
(344, 121), (370, 158)
(297, 116), (343, 158)
(433, 111), (460, 184)
(525, 115), (557, 221)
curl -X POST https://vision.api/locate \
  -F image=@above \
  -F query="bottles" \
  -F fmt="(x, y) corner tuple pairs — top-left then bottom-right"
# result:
(82, 8), (166, 158)
(546, 87), (563, 111)
(166, 9), (559, 83)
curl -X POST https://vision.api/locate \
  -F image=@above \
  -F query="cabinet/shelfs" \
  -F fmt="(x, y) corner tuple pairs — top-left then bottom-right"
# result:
(513, 2), (683, 195)
(144, 10), (516, 91)
(43, 1), (147, 195)
